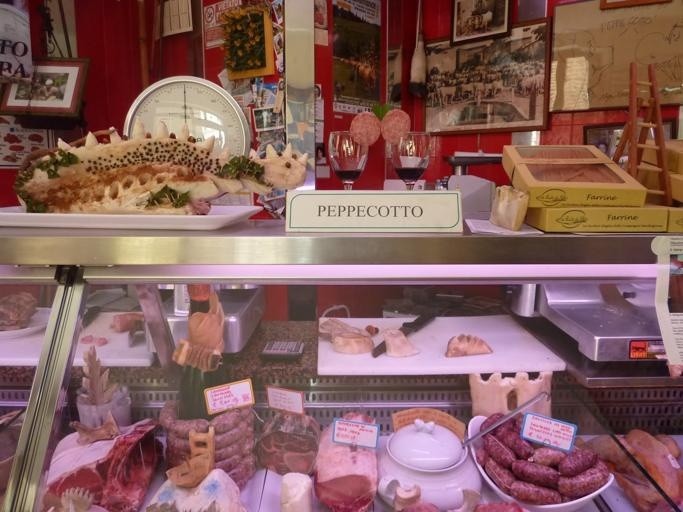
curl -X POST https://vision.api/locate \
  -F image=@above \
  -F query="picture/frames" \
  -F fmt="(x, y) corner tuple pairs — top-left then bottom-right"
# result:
(1, 56), (90, 119)
(420, 0), (552, 136)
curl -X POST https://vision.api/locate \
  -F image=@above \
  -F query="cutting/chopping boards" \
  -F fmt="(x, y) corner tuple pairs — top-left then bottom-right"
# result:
(0, 303), (155, 368)
(315, 314), (568, 378)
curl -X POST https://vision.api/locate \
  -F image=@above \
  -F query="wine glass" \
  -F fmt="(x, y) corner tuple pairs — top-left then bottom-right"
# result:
(326, 128), (371, 190)
(388, 128), (436, 191)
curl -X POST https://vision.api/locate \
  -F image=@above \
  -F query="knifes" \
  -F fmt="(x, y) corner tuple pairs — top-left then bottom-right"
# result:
(369, 309), (437, 359)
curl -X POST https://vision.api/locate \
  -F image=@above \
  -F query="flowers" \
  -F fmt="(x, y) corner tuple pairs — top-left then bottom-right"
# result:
(217, 6), (266, 71)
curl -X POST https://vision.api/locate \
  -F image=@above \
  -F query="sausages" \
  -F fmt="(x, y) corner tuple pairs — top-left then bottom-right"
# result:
(158, 403), (255, 492)
(477, 412), (608, 505)
(112, 314), (145, 334)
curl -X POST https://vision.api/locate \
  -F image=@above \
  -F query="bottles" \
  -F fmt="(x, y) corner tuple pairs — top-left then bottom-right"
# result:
(175, 278), (233, 422)
(373, 417), (481, 511)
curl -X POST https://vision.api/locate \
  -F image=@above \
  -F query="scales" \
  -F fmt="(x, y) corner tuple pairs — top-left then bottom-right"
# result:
(122, 76), (264, 360)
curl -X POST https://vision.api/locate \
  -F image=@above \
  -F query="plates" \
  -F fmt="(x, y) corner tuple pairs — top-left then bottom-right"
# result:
(465, 411), (617, 512)
(1, 200), (264, 232)
(0, 305), (52, 340)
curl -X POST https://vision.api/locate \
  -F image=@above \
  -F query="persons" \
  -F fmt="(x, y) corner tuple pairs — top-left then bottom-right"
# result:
(316, 146), (327, 165)
(596, 142), (608, 154)
(39, 79), (58, 100)
(260, 109), (272, 128)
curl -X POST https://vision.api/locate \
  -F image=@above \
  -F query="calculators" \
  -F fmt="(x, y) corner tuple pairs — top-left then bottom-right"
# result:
(260, 342), (304, 357)
